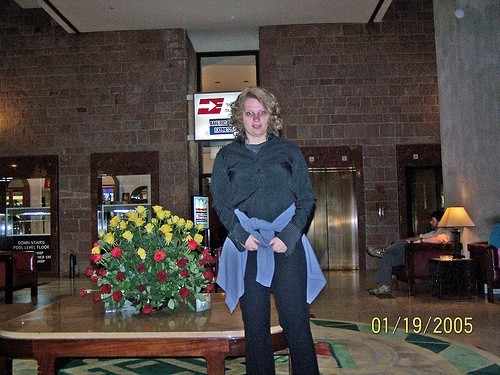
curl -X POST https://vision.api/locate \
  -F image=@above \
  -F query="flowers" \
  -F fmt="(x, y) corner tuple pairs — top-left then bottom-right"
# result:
(78, 205), (216, 314)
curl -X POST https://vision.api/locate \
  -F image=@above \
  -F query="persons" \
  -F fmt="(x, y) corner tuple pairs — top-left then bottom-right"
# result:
(210, 87), (327, 375)
(367, 209), (453, 296)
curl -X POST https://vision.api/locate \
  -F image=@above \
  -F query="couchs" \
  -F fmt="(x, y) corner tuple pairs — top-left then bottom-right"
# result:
(465, 239), (500, 303)
(392, 228), (461, 296)
(0, 249), (39, 305)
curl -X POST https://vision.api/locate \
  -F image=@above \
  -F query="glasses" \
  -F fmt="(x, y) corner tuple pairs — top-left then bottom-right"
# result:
(243, 110), (269, 117)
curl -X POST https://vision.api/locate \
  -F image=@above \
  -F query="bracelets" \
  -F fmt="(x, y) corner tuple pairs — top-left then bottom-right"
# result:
(420, 237), (424, 243)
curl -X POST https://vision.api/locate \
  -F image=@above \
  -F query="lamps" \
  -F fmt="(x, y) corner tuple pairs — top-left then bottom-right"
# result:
(437, 207), (476, 259)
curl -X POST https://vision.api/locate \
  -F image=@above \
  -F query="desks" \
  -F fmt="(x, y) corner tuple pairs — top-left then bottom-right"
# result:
(0, 290), (289, 375)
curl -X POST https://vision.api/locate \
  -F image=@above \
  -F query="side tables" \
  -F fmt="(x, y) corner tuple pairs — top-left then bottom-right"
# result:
(429, 257), (484, 300)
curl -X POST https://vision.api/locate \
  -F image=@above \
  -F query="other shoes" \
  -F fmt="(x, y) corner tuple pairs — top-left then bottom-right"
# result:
(366, 245), (383, 259)
(368, 284), (393, 296)
